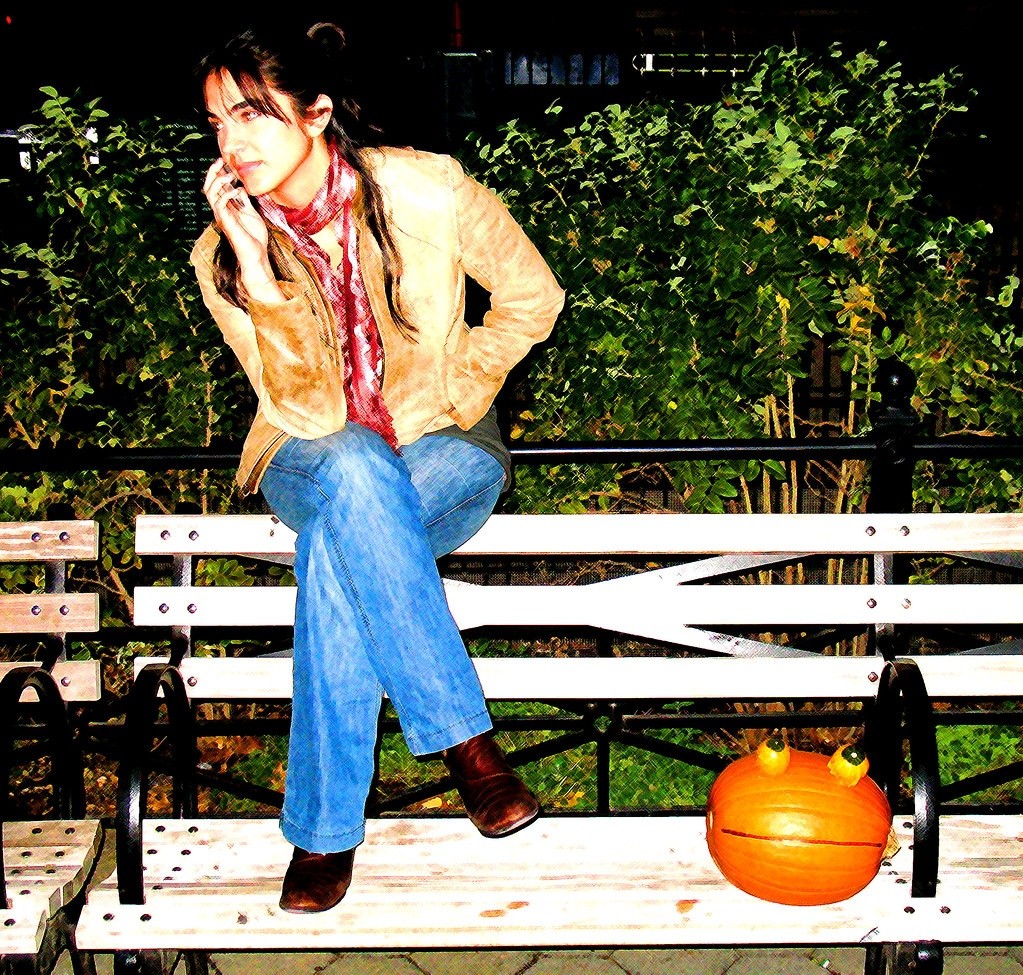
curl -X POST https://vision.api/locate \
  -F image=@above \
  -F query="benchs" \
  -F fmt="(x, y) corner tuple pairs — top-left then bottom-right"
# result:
(1, 500), (103, 975)
(73, 500), (1023, 975)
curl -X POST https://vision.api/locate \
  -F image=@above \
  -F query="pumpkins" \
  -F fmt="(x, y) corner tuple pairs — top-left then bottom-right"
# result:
(706, 740), (902, 907)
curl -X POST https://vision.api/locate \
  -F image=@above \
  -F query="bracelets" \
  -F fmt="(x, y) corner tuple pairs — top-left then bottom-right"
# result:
(241, 276), (275, 287)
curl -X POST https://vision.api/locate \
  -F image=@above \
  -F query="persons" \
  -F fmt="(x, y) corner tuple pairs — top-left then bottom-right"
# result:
(188, 22), (566, 913)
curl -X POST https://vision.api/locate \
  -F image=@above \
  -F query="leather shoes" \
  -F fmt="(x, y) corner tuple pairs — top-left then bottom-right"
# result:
(440, 732), (541, 838)
(278, 843), (355, 914)
